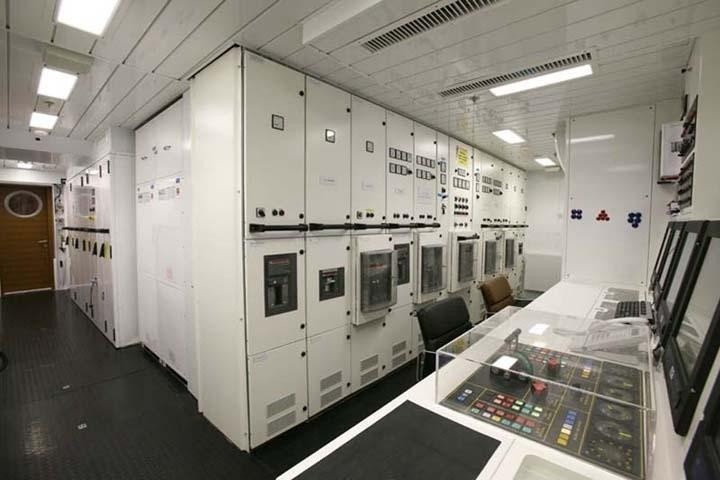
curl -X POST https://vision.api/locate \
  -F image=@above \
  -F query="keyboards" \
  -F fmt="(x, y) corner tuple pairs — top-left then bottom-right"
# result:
(613, 299), (653, 322)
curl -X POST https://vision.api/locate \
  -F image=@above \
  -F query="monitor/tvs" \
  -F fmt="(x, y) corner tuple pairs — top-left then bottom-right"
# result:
(650, 220), (720, 480)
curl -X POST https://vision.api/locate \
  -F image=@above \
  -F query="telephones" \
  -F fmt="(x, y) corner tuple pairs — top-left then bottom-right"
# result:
(581, 315), (651, 352)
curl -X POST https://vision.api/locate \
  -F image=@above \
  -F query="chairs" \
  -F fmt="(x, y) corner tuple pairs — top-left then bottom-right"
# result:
(416, 296), (477, 382)
(478, 273), (533, 321)
